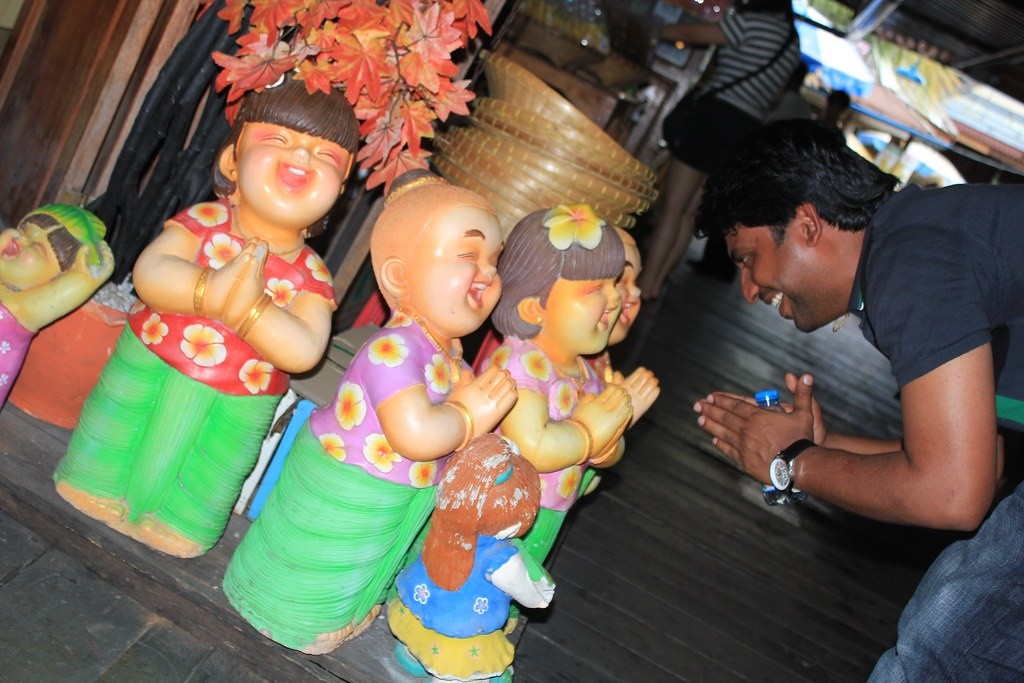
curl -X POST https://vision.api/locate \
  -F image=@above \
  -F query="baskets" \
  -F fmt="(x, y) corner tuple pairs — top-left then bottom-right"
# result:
(434, 46), (662, 241)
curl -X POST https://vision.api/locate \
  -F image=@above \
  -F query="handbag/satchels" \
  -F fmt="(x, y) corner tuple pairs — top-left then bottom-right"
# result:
(662, 90), (701, 147)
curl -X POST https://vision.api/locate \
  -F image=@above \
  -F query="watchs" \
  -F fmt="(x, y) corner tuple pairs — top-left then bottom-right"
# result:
(769, 437), (819, 497)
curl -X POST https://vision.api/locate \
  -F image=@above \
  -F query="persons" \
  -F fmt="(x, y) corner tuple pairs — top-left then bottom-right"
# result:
(0, 68), (662, 683)
(635, 1), (803, 301)
(802, 88), (852, 148)
(690, 115), (1023, 683)
(686, 58), (808, 286)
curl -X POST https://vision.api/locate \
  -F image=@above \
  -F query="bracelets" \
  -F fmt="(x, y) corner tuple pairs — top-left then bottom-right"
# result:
(235, 293), (272, 340)
(562, 417), (593, 467)
(441, 401), (473, 454)
(193, 267), (213, 316)
(589, 440), (620, 465)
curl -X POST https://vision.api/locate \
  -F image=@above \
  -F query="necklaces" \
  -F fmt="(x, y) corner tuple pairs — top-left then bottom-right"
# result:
(397, 306), (465, 385)
(529, 342), (588, 403)
(231, 206), (306, 257)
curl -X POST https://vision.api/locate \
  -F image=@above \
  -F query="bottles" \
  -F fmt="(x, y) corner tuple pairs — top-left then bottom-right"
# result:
(755, 389), (809, 506)
